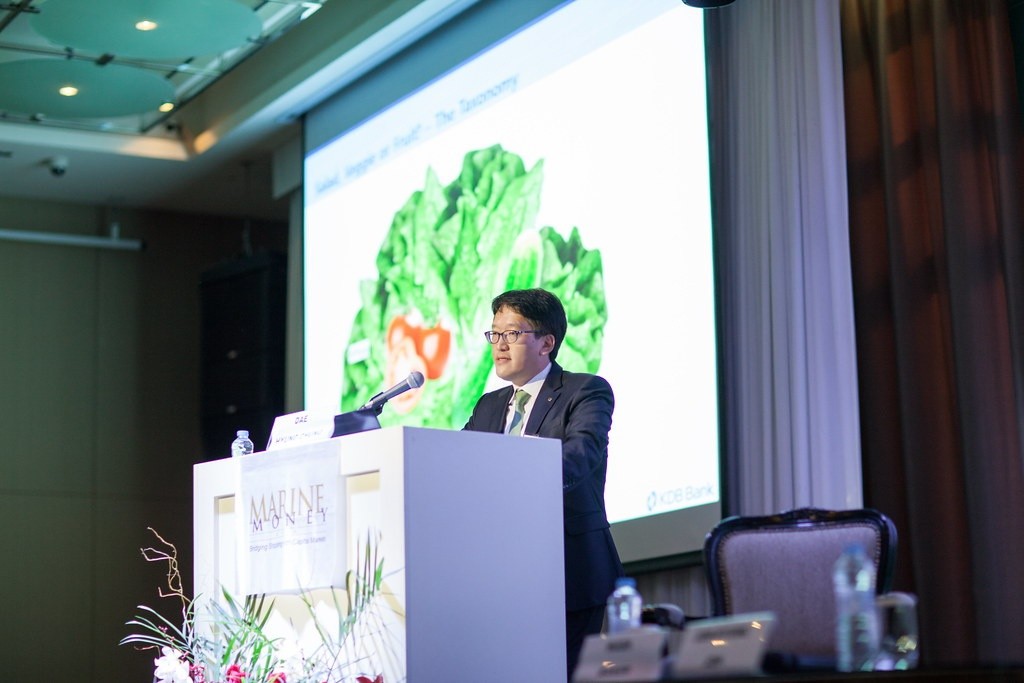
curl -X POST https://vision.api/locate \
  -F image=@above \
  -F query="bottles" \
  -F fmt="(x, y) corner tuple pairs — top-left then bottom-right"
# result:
(605, 577), (643, 636)
(829, 544), (880, 670)
(231, 431), (254, 457)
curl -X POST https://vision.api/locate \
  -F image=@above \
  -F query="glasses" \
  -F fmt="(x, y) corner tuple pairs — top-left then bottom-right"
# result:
(484, 329), (540, 344)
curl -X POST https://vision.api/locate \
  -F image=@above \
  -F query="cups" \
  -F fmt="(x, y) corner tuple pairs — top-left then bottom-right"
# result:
(876, 592), (921, 670)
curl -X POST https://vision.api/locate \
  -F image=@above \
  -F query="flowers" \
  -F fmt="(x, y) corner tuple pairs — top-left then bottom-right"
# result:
(118, 526), (404, 683)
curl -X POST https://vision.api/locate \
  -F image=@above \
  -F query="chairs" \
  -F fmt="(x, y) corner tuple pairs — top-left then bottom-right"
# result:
(641, 505), (897, 657)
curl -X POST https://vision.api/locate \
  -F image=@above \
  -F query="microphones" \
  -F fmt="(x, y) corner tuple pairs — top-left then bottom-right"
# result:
(363, 372), (424, 410)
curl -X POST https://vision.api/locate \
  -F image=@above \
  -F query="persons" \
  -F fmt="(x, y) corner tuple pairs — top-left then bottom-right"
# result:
(458, 289), (625, 683)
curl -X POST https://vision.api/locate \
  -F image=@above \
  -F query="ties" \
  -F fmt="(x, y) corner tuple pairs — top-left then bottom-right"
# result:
(505, 390), (532, 435)
